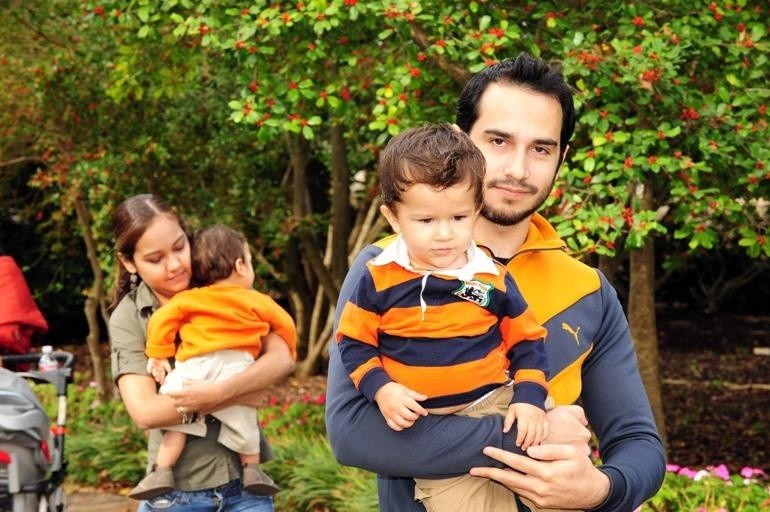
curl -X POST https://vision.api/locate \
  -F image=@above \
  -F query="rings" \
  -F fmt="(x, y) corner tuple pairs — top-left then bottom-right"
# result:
(176, 407), (184, 415)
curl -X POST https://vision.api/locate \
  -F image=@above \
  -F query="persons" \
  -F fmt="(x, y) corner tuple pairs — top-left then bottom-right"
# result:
(325, 51), (665, 512)
(128, 224), (298, 500)
(108, 192), (296, 512)
(335, 123), (584, 512)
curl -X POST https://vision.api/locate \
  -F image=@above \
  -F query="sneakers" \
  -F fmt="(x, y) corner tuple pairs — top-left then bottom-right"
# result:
(128, 470), (174, 500)
(241, 463), (281, 495)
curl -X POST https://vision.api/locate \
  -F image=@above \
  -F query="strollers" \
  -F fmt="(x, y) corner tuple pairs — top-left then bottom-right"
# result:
(0, 352), (74, 512)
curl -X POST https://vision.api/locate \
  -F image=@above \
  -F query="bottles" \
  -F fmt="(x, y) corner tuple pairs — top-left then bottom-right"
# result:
(37, 345), (60, 371)
